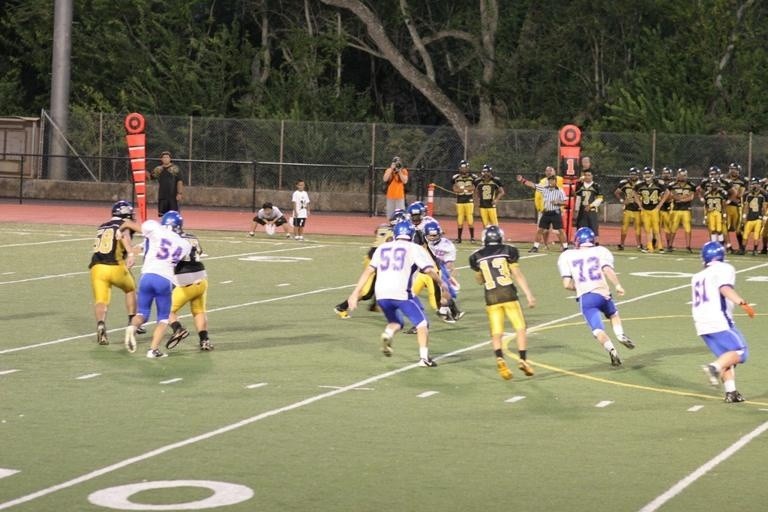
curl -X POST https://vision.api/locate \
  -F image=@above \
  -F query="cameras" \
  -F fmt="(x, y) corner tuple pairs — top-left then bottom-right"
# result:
(395, 161), (403, 172)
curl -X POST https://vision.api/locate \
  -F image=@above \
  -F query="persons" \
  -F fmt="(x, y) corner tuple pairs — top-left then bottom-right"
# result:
(90, 199), (147, 345)
(119, 218), (190, 359)
(334, 200), (465, 368)
(474, 164), (505, 228)
(452, 159), (477, 245)
(469, 224), (537, 380)
(291, 178), (310, 240)
(249, 201), (290, 239)
(690, 241), (756, 404)
(382, 156), (408, 226)
(161, 210), (215, 351)
(145, 151), (185, 221)
(556, 227), (634, 368)
(516, 161), (768, 256)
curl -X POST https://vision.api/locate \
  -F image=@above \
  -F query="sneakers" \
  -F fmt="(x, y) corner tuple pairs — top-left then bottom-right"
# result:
(98, 322), (214, 358)
(381, 334), (393, 357)
(334, 304), (351, 318)
(437, 308), (455, 324)
(419, 358), (437, 367)
(704, 365), (718, 386)
(527, 246), (538, 254)
(453, 237), (476, 244)
(618, 244), (692, 253)
(610, 338), (635, 365)
(724, 390), (745, 403)
(496, 357), (533, 379)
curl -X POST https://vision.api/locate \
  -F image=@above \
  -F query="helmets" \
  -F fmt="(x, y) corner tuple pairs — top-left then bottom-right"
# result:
(708, 162), (740, 177)
(575, 227), (595, 247)
(483, 225), (505, 245)
(161, 210), (182, 229)
(112, 200), (137, 222)
(630, 166), (688, 178)
(390, 201), (440, 241)
(459, 160), (493, 179)
(702, 242), (724, 265)
